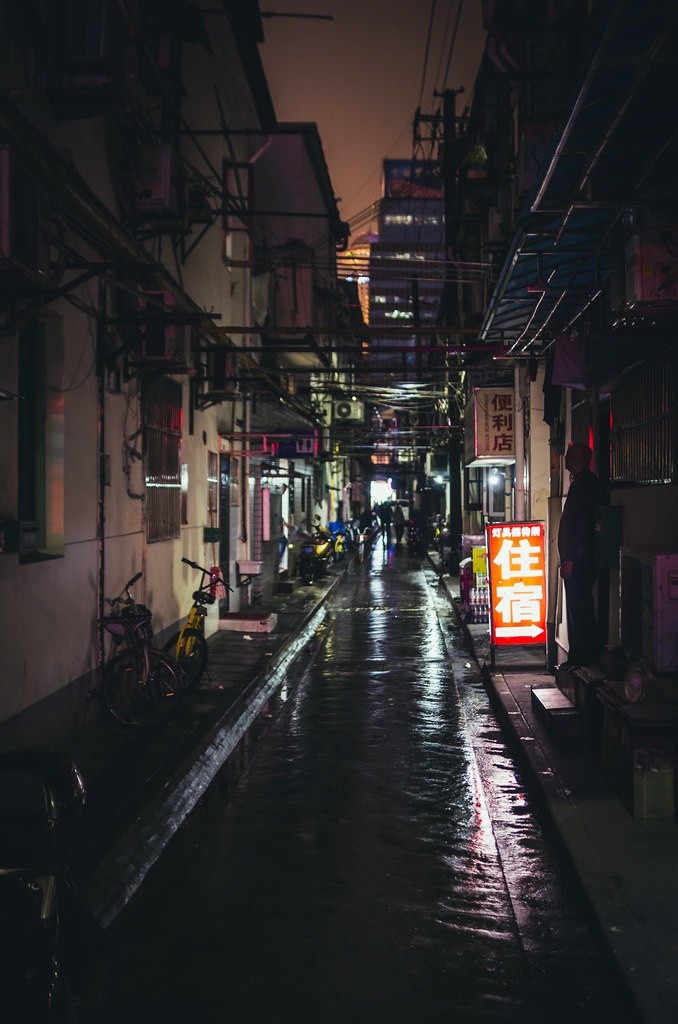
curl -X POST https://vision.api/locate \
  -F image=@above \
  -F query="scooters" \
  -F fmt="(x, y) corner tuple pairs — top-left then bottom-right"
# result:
(296, 514), (365, 584)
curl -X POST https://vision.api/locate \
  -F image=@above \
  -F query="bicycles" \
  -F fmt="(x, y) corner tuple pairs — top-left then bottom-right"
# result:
(156, 557), (233, 700)
(98, 572), (182, 728)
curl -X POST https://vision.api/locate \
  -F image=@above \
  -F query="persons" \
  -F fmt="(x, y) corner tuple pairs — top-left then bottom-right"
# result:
(373, 501), (405, 543)
(558, 443), (601, 676)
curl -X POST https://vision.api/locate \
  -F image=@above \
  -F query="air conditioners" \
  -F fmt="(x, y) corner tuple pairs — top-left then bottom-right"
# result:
(609, 201), (678, 326)
(617, 546), (677, 681)
(332, 400), (363, 421)
(135, 140), (189, 232)
(206, 344), (239, 395)
(139, 291), (193, 374)
(516, 122), (556, 197)
